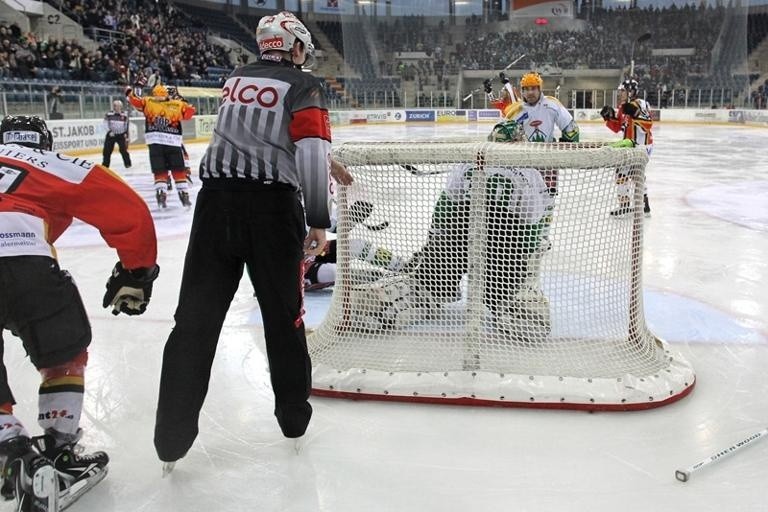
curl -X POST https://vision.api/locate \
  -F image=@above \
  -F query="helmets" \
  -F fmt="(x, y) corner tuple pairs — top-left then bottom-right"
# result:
(255, 9), (319, 69)
(617, 80), (640, 101)
(1, 114), (53, 151)
(152, 85), (168, 99)
(489, 119), (527, 143)
(521, 72), (543, 104)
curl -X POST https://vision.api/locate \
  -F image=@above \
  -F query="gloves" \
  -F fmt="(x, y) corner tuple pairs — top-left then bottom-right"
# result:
(499, 72), (509, 83)
(619, 101), (638, 120)
(601, 105), (615, 121)
(103, 262), (162, 317)
(483, 78), (492, 94)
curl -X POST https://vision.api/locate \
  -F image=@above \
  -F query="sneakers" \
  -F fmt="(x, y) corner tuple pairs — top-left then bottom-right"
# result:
(157, 189), (168, 206)
(610, 201), (631, 216)
(180, 193), (193, 205)
(49, 432), (108, 491)
(1, 437), (60, 512)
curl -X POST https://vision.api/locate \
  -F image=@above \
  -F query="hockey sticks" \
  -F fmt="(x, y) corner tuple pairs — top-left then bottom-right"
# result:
(331, 199), (387, 230)
(402, 162), (455, 177)
(622, 33), (652, 130)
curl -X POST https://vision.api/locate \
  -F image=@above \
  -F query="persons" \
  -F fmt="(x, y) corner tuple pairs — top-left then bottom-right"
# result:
(47, 85), (65, 120)
(154, 11), (332, 476)
(126, 85), (194, 209)
(0, 0), (235, 82)
(302, 201), (412, 293)
(504, 73), (579, 249)
(484, 4), (625, 70)
(375, 13), (484, 106)
(1, 114), (160, 511)
(484, 71), (520, 119)
(345, 120), (548, 343)
(102, 100), (132, 169)
(658, 6), (728, 105)
(625, 7), (657, 79)
(600, 79), (654, 217)
